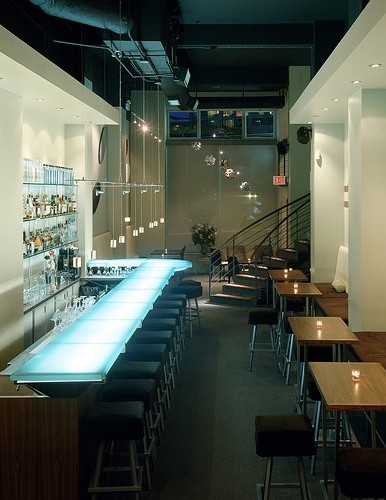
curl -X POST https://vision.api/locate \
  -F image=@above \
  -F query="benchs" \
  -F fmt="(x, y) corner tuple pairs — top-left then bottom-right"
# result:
(347, 331), (386, 370)
(335, 446), (386, 500)
(312, 282), (348, 326)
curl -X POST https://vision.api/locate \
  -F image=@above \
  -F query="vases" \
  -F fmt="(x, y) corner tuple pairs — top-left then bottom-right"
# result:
(200, 244), (208, 257)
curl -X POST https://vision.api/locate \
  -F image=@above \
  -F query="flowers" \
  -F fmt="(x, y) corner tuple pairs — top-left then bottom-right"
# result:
(191, 223), (217, 248)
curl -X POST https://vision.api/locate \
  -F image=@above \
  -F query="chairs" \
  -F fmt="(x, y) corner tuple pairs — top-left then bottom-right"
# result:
(209, 245), (274, 284)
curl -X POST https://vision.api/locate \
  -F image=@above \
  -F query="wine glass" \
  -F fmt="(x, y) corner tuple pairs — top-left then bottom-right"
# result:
(51, 296), (96, 337)
(24, 274), (45, 311)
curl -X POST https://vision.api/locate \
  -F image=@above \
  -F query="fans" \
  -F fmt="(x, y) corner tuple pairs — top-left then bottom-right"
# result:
(297, 126), (313, 145)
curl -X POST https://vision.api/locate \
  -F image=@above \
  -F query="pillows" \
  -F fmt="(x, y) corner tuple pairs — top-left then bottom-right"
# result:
(332, 246), (348, 293)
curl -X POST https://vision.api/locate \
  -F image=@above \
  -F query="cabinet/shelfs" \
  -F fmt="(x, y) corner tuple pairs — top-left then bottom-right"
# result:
(22, 182), (82, 350)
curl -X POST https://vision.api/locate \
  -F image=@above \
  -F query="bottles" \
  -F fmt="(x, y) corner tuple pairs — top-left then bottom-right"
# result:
(55, 271), (61, 288)
(87, 264), (133, 276)
(23, 219), (77, 256)
(23, 193), (76, 221)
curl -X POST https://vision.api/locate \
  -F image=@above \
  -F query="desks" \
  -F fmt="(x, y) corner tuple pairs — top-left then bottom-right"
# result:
(193, 257), (210, 276)
(275, 282), (323, 327)
(307, 361), (386, 496)
(287, 316), (360, 399)
(268, 270), (308, 308)
(221, 260), (264, 281)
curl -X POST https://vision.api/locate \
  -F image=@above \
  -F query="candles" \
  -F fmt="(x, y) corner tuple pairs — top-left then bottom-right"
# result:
(317, 320), (323, 329)
(352, 369), (360, 381)
(284, 269), (288, 273)
(289, 268), (293, 272)
(293, 283), (298, 288)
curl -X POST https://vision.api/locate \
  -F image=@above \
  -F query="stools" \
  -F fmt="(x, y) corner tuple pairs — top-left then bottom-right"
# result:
(273, 298), (353, 448)
(255, 413), (317, 500)
(247, 311), (279, 372)
(80, 279), (204, 500)
